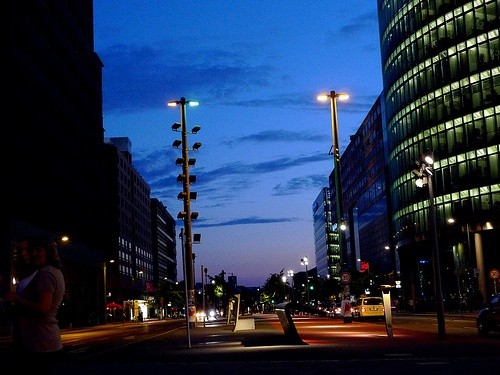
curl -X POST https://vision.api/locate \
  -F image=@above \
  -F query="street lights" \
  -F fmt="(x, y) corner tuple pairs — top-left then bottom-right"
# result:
(166, 96), (203, 329)
(316, 89), (353, 285)
(414, 156), (451, 340)
(300, 255), (311, 299)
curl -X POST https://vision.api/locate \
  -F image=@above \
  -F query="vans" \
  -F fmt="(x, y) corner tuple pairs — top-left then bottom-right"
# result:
(350, 296), (385, 321)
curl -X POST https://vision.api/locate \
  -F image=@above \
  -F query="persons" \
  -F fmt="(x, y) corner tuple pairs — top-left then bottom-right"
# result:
(0, 235), (64, 375)
